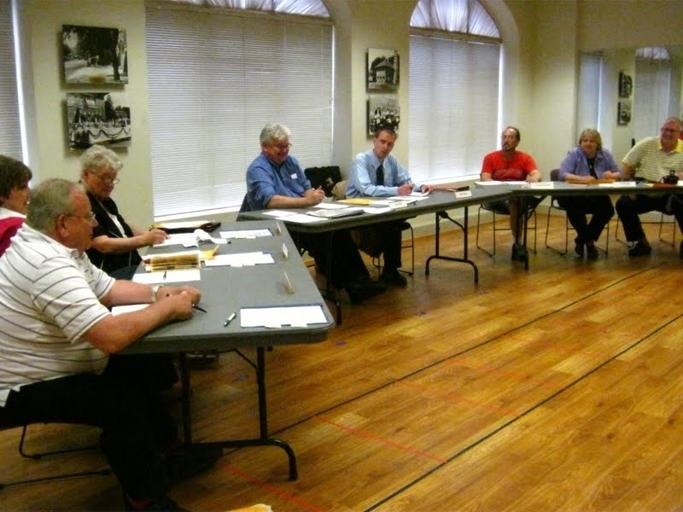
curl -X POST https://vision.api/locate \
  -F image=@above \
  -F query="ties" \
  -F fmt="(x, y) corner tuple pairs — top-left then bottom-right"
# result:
(376, 161), (385, 186)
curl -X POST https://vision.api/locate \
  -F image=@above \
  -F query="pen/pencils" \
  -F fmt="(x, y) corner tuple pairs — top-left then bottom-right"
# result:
(407, 180), (415, 192)
(148, 224), (169, 240)
(166, 293), (209, 314)
(162, 270), (166, 279)
(224, 312), (236, 327)
(312, 187), (328, 200)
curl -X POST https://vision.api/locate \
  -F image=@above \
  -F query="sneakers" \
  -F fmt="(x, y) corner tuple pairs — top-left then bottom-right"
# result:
(512, 243), (528, 261)
(348, 279), (387, 304)
(587, 244), (599, 258)
(383, 268), (408, 286)
(166, 439), (225, 473)
(124, 494), (192, 511)
(628, 242), (653, 256)
(574, 238), (585, 255)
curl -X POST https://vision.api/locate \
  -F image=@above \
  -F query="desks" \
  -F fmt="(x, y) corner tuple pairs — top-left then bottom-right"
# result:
(238, 187), (513, 325)
(112, 220), (335, 479)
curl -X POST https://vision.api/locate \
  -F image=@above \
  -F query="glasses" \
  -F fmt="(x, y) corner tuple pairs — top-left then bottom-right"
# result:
(276, 143), (292, 149)
(67, 213), (96, 223)
(89, 170), (121, 186)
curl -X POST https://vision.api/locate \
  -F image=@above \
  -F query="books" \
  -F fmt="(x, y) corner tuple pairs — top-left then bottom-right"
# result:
(429, 182), (471, 191)
(146, 253), (199, 270)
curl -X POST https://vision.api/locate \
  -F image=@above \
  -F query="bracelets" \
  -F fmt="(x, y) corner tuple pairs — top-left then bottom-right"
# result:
(149, 284), (162, 302)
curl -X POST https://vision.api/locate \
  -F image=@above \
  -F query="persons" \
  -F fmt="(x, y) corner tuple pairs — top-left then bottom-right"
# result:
(0, 177), (224, 511)
(556, 127), (623, 260)
(104, 93), (118, 122)
(236, 121), (386, 305)
(615, 115), (683, 259)
(0, 155), (33, 257)
(73, 109), (85, 122)
(73, 145), (169, 281)
(345, 125), (436, 288)
(479, 125), (541, 262)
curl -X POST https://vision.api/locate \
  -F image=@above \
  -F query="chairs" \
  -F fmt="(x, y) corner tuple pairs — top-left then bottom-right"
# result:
(476, 169), (683, 257)
(332, 180), (413, 283)
(0, 354), (190, 488)
(244, 194), (330, 295)
